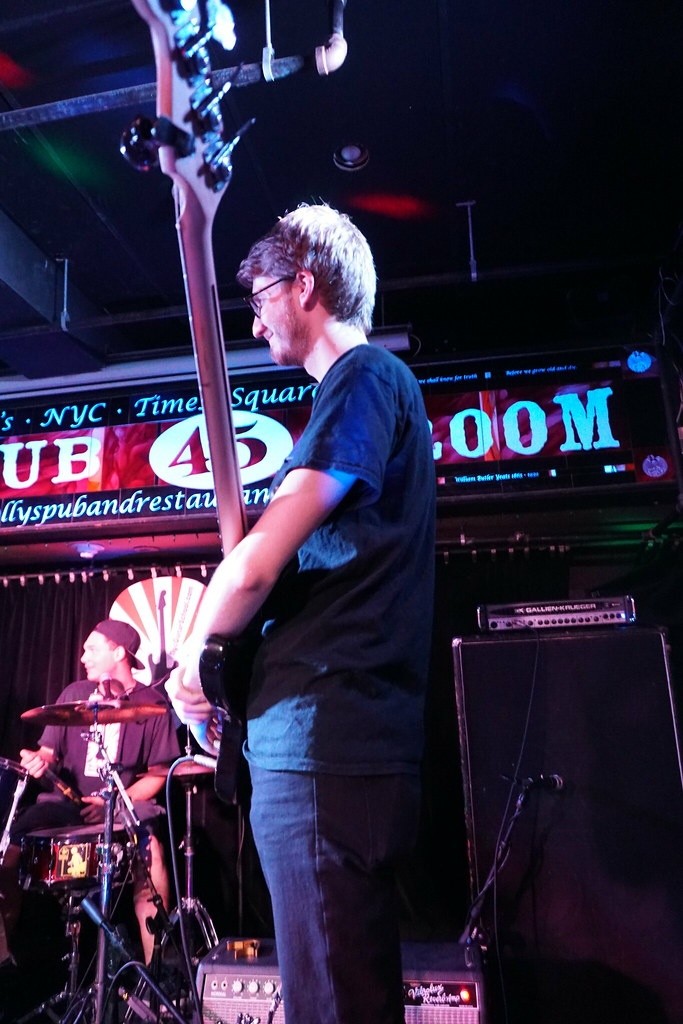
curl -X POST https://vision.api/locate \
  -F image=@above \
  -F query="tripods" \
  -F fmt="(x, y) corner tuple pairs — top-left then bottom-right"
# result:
(10, 709), (222, 1024)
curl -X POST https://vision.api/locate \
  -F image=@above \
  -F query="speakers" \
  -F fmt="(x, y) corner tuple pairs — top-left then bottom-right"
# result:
(451, 626), (683, 1024)
(191, 935), (486, 1024)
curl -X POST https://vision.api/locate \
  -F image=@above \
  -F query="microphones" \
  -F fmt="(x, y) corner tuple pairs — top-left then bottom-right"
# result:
(523, 774), (564, 792)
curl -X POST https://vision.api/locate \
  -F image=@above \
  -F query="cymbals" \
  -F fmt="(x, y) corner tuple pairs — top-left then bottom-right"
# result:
(21, 699), (167, 724)
(134, 759), (214, 784)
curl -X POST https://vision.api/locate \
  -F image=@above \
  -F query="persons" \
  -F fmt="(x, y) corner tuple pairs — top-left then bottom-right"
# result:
(19, 619), (182, 968)
(165, 204), (439, 1024)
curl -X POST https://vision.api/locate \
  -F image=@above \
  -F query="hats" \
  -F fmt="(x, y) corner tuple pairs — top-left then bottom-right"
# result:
(93, 620), (146, 670)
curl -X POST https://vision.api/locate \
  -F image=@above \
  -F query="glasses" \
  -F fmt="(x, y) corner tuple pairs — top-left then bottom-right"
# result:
(241, 276), (289, 318)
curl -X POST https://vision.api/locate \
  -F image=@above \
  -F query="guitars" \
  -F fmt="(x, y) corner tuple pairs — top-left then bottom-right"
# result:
(116, 0), (259, 808)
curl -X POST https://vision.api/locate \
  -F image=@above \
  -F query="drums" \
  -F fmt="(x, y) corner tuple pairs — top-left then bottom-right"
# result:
(19, 823), (130, 895)
